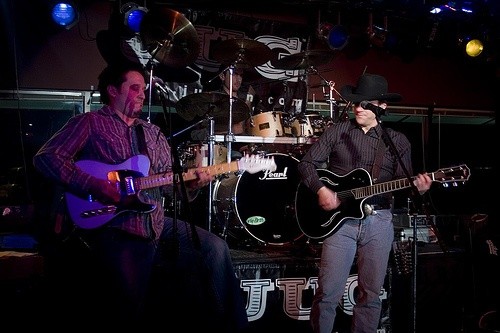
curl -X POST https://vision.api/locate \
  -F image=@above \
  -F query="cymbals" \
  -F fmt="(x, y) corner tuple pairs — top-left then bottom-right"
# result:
(273, 48), (336, 71)
(139, 7), (199, 70)
(211, 38), (272, 70)
(174, 91), (249, 124)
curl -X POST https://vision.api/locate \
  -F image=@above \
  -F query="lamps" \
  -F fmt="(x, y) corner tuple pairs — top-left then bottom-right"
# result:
(367, 22), (387, 46)
(446, 1), (459, 12)
(318, 19), (353, 52)
(125, 6), (150, 34)
(49, 0), (79, 29)
(428, 3), (441, 14)
(453, 31), (484, 58)
(461, 1), (473, 14)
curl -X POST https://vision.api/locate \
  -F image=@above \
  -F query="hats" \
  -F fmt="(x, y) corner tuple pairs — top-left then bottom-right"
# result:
(341, 74), (401, 104)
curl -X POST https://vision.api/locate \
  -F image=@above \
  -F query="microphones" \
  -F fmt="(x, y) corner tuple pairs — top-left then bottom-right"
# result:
(144, 70), (167, 95)
(361, 101), (388, 116)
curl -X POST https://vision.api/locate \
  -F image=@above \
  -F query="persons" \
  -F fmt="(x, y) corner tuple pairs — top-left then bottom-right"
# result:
(298, 74), (413, 333)
(214, 62), (255, 109)
(34, 61), (249, 333)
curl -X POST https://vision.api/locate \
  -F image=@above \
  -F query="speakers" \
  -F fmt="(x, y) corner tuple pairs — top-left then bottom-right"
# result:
(389, 249), (472, 333)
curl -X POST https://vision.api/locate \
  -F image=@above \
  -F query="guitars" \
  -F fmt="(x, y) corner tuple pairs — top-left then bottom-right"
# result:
(63, 154), (278, 230)
(294, 161), (471, 242)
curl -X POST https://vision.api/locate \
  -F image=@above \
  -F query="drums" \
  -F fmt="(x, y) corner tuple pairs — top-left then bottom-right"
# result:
(287, 111), (325, 153)
(181, 143), (227, 169)
(211, 153), (301, 248)
(246, 111), (292, 152)
